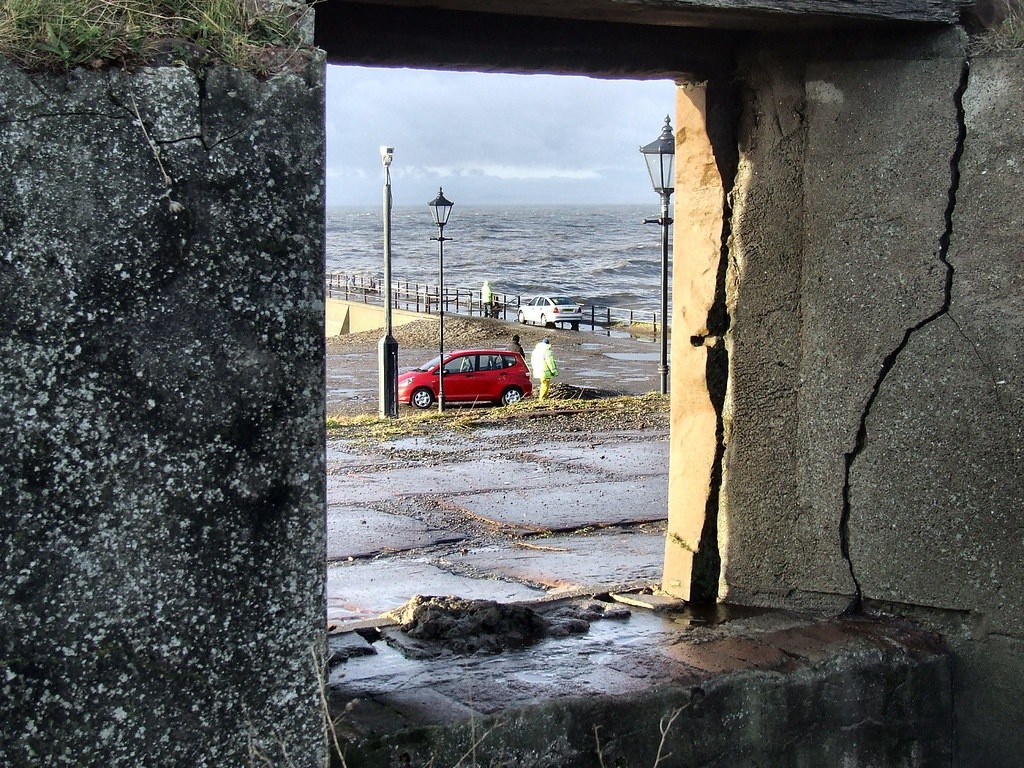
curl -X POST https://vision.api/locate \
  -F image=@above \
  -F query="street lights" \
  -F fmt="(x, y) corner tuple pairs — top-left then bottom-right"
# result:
(378, 145), (399, 422)
(427, 187), (454, 413)
(639, 114), (676, 394)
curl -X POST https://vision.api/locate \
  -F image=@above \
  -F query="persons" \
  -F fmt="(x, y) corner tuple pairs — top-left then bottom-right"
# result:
(507, 335), (525, 361)
(351, 274), (376, 291)
(531, 339), (558, 399)
(482, 279), (503, 318)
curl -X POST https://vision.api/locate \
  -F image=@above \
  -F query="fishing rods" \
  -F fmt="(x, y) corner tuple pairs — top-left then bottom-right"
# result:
(501, 278), (546, 308)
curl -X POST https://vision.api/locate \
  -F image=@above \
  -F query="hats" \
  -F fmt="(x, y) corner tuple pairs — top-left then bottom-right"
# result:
(543, 339), (549, 344)
(513, 335), (519, 341)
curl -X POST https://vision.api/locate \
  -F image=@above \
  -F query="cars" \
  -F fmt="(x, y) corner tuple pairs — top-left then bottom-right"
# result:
(399, 348), (533, 410)
(516, 294), (583, 330)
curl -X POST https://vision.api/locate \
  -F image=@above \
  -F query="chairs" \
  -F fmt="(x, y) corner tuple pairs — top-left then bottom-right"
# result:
(462, 358), (473, 371)
(460, 356), (468, 372)
